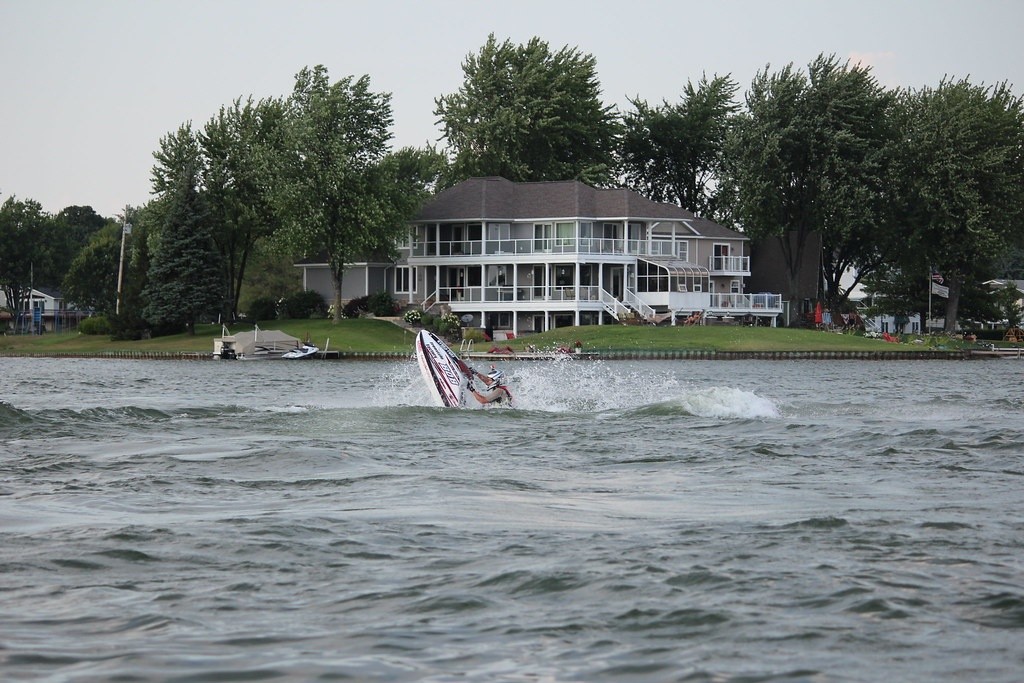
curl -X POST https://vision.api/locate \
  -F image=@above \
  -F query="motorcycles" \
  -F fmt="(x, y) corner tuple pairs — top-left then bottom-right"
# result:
(415, 329), (488, 411)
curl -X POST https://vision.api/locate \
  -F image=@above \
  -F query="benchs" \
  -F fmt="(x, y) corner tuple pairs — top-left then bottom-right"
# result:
(617, 313), (643, 326)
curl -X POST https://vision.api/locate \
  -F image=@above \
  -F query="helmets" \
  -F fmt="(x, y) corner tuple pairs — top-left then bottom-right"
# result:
(486, 370), (505, 391)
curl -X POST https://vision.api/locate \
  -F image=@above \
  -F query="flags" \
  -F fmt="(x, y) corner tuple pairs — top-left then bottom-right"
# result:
(932, 281), (950, 298)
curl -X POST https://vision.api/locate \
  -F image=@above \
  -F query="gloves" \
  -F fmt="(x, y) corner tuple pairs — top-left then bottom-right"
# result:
(467, 382), (476, 392)
(469, 367), (477, 374)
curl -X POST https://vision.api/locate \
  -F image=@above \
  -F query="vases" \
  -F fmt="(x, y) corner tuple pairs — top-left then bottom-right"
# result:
(412, 320), (422, 327)
(575, 348), (582, 353)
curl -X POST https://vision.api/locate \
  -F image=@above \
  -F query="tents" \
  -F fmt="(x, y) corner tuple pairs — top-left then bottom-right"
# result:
(232, 329), (304, 353)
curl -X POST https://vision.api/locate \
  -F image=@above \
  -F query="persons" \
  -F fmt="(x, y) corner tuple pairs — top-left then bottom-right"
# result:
(963, 331), (967, 337)
(815, 300), (822, 330)
(467, 367), (514, 408)
(821, 308), (831, 333)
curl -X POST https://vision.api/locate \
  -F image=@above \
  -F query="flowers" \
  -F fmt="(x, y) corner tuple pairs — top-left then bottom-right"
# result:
(576, 341), (582, 348)
(403, 310), (422, 325)
